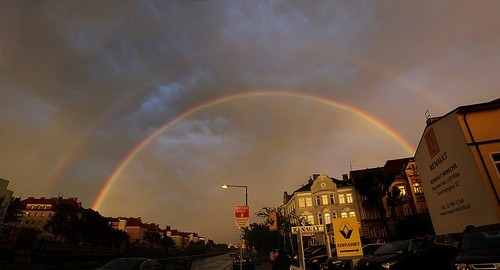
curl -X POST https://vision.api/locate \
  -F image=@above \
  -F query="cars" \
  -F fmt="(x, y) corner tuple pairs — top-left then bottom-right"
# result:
(356, 235), (458, 270)
(290, 237), (387, 270)
(96, 257), (165, 270)
(456, 224), (500, 270)
(160, 251), (193, 270)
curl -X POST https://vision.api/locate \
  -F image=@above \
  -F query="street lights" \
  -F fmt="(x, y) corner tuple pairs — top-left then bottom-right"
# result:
(221, 184), (249, 266)
